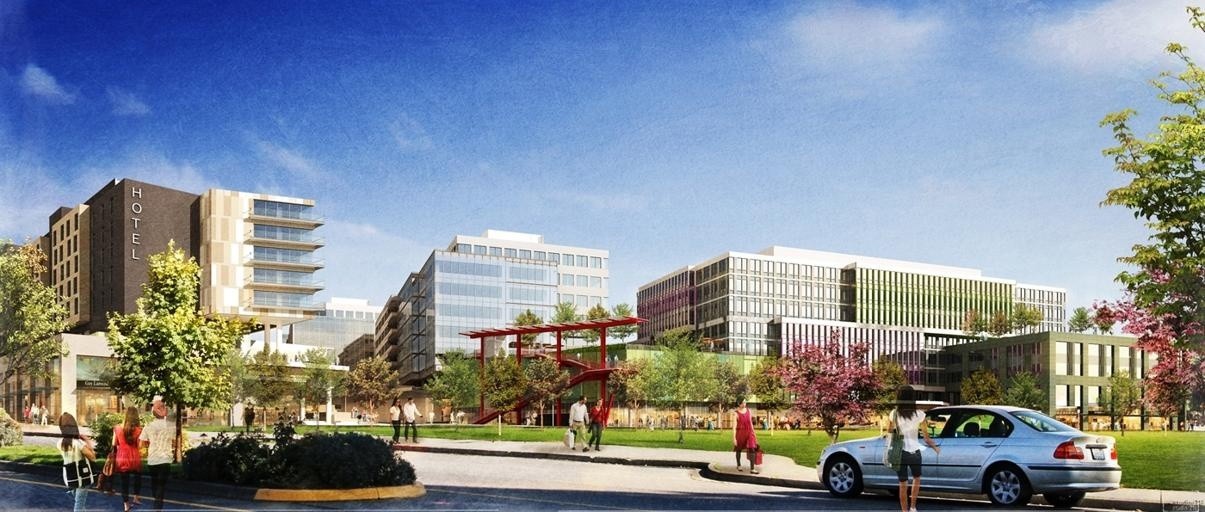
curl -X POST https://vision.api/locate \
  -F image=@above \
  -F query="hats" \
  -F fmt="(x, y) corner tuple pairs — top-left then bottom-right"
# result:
(152, 399), (169, 417)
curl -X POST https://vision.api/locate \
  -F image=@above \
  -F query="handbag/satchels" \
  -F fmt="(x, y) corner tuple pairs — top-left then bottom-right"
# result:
(883, 410), (905, 472)
(62, 440), (100, 489)
(103, 444), (117, 478)
(562, 429), (576, 450)
(746, 444), (763, 466)
